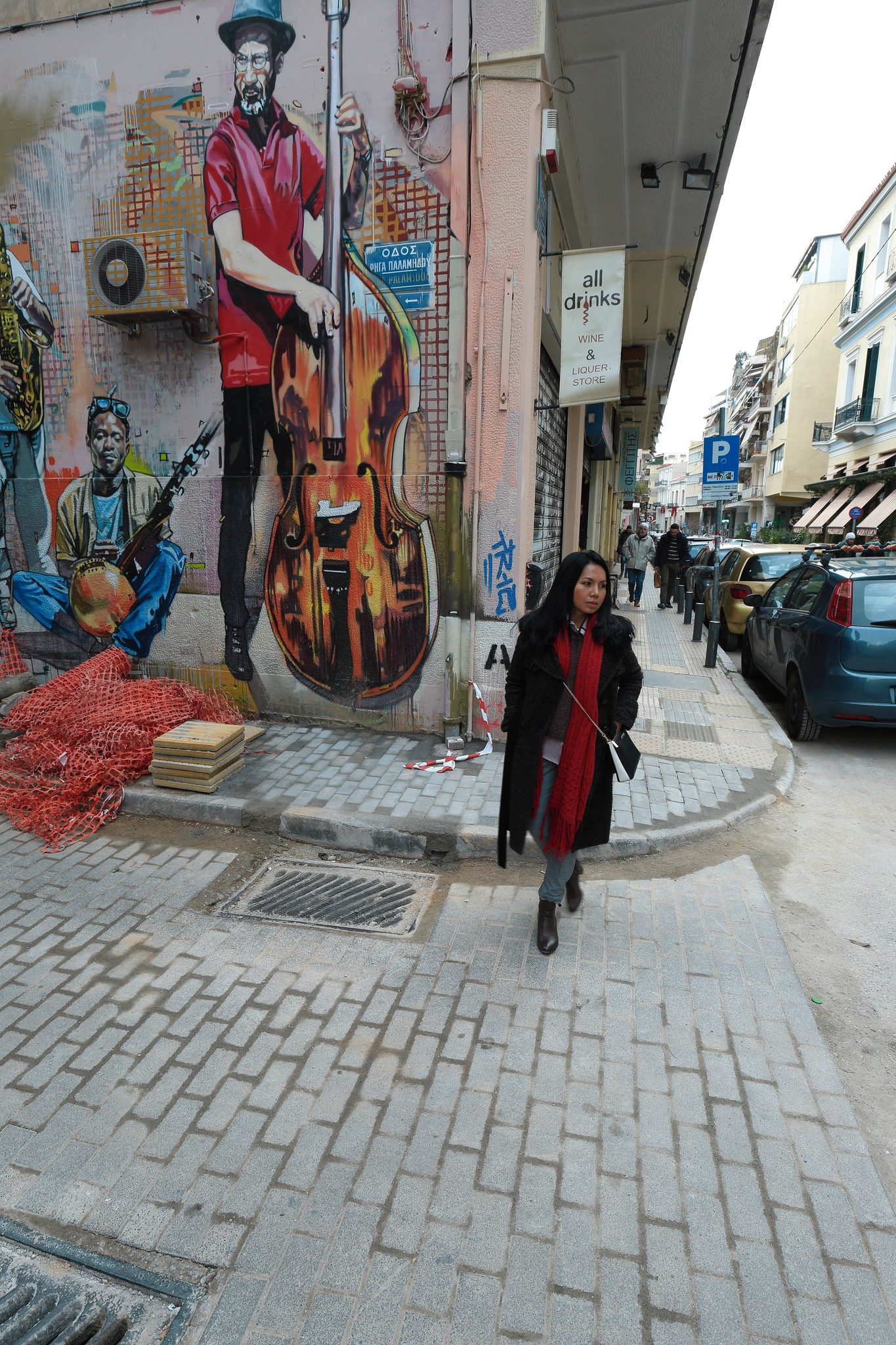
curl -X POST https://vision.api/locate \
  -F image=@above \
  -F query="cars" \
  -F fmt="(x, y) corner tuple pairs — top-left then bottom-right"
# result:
(646, 530), (669, 553)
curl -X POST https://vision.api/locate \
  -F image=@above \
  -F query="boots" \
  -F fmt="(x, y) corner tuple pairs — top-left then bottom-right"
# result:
(536, 899), (559, 954)
(565, 859), (584, 912)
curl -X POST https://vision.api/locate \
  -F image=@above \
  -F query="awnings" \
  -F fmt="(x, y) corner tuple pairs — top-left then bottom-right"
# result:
(739, 430), (745, 439)
(825, 464), (847, 478)
(808, 484), (859, 533)
(740, 412), (760, 449)
(820, 473), (827, 480)
(856, 488), (896, 536)
(827, 479), (889, 534)
(866, 452), (896, 468)
(793, 486), (841, 533)
(843, 460), (869, 473)
(704, 412), (719, 429)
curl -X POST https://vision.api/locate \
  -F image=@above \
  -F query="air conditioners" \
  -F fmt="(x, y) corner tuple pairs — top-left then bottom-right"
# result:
(755, 489), (762, 497)
(82, 228), (209, 323)
(779, 336), (786, 347)
(744, 468), (750, 475)
(766, 430), (773, 439)
(740, 470), (745, 477)
(767, 371), (773, 381)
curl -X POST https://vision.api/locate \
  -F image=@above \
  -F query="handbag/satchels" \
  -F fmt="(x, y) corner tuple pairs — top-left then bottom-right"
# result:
(654, 570), (661, 588)
(604, 730), (641, 783)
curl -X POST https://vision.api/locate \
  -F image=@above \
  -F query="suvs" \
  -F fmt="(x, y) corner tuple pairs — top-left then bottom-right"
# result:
(685, 532), (838, 651)
(740, 544), (896, 742)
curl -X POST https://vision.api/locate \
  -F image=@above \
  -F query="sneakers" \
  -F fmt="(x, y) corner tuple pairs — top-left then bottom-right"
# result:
(658, 603), (666, 609)
(666, 603), (673, 608)
(634, 602), (640, 606)
(629, 594), (634, 602)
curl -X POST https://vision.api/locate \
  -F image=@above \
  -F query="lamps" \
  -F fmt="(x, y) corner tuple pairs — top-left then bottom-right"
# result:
(641, 165), (660, 188)
(666, 329), (675, 346)
(679, 266), (690, 287)
(683, 153), (714, 191)
(649, 385), (668, 457)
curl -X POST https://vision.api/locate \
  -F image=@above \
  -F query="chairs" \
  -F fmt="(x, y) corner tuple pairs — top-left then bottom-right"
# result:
(752, 561), (770, 574)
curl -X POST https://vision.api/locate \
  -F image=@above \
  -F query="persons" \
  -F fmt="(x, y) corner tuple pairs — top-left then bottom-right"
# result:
(622, 522), (656, 607)
(654, 523), (689, 609)
(860, 534), (885, 558)
(616, 525), (638, 578)
(835, 532), (856, 558)
(496, 547), (644, 956)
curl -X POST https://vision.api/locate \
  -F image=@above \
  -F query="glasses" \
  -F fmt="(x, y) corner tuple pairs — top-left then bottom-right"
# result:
(640, 528), (647, 530)
(670, 531), (678, 534)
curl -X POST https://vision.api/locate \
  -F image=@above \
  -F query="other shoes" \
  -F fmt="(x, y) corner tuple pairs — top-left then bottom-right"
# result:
(624, 574), (627, 578)
(620, 572), (623, 577)
